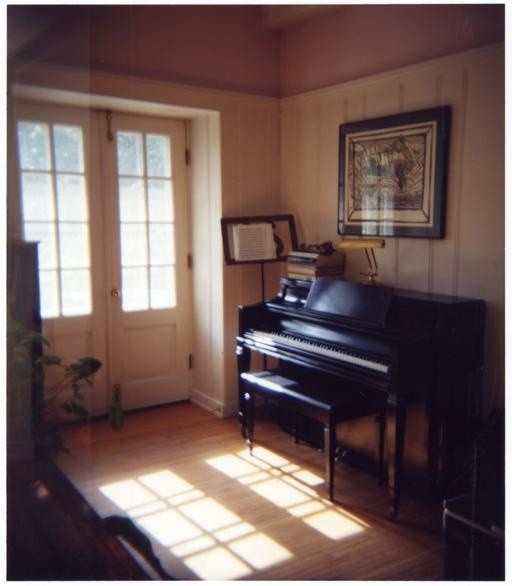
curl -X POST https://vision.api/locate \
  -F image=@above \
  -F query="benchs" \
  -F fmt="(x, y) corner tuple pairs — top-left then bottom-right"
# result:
(239, 364), (388, 501)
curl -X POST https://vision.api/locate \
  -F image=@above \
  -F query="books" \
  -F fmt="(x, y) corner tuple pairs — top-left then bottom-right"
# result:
(287, 250), (346, 282)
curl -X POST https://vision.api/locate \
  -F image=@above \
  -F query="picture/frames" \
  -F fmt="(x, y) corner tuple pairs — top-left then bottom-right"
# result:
(337, 105), (450, 240)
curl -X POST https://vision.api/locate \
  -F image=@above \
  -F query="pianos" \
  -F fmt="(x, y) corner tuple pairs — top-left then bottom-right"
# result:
(236, 278), (487, 523)
